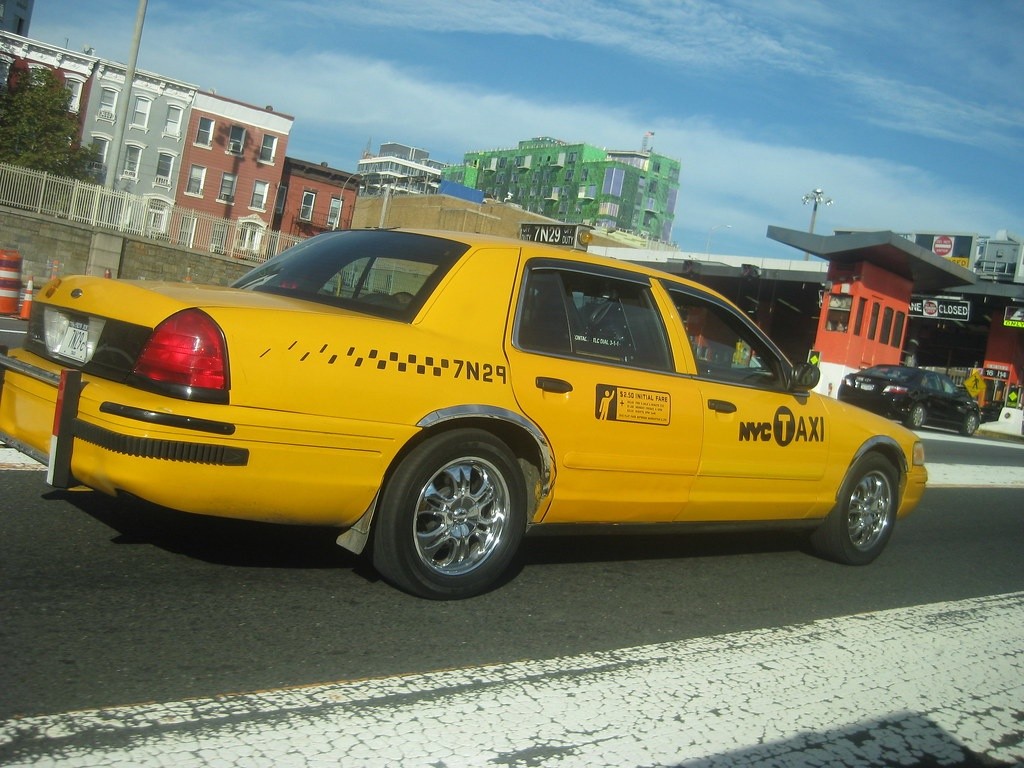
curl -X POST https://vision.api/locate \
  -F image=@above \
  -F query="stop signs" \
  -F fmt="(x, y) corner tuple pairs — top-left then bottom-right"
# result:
(923, 299), (938, 316)
(932, 236), (954, 257)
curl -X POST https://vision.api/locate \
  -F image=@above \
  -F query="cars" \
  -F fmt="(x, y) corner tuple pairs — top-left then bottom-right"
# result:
(838, 364), (981, 437)
(693, 343), (761, 369)
(980, 400), (1004, 424)
(0, 229), (929, 600)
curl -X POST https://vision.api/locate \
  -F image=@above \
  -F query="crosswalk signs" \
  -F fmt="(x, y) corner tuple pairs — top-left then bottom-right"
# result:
(963, 372), (986, 398)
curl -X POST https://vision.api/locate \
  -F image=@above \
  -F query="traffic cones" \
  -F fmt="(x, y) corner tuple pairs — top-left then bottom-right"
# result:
(16, 275), (33, 320)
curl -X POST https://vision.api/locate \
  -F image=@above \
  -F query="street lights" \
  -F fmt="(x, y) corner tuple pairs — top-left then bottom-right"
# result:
(707, 224), (731, 260)
(332, 173), (360, 230)
(802, 188), (834, 261)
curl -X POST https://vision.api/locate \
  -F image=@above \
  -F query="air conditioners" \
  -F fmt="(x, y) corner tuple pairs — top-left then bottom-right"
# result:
(228, 142), (242, 153)
(209, 244), (220, 253)
